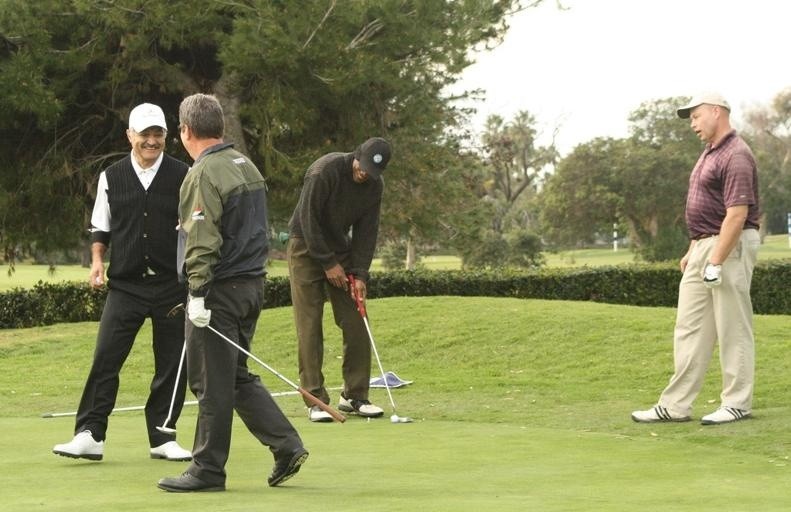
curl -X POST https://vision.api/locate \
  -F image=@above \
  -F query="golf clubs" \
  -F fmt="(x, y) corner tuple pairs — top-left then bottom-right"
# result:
(166, 303), (345, 424)
(348, 274), (414, 424)
(155, 336), (189, 433)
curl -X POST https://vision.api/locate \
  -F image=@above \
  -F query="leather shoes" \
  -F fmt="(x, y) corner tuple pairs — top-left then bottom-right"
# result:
(149, 441), (192, 462)
(267, 447), (310, 488)
(53, 433), (105, 460)
(157, 471), (226, 493)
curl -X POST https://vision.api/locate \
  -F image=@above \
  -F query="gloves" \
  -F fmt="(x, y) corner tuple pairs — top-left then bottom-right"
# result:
(703, 263), (724, 287)
(187, 299), (212, 328)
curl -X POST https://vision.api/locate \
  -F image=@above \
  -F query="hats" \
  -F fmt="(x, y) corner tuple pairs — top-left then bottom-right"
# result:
(128, 102), (167, 134)
(677, 91), (732, 119)
(358, 135), (392, 182)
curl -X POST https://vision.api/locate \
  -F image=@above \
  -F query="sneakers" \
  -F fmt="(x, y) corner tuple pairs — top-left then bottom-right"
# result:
(338, 391), (384, 418)
(702, 405), (752, 425)
(631, 403), (691, 423)
(307, 405), (333, 422)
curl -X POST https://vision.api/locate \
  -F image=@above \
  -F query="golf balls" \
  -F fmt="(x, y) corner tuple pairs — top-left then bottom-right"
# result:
(389, 416), (398, 422)
(96, 274), (107, 284)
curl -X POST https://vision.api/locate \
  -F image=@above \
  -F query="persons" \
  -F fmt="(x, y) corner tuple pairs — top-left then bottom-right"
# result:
(158, 89), (307, 494)
(50, 102), (193, 463)
(287, 137), (392, 422)
(632, 96), (760, 426)
